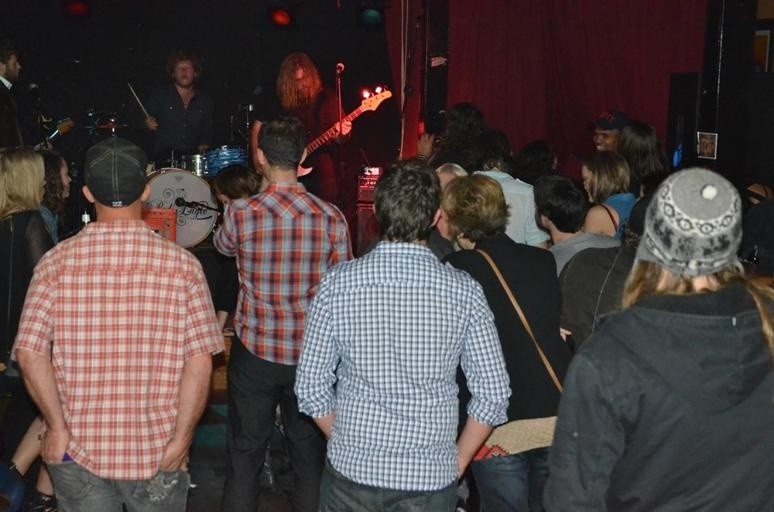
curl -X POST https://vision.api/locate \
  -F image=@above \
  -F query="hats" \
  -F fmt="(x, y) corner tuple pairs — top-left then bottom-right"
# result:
(587, 112), (625, 131)
(85, 137), (147, 207)
(637, 167), (744, 277)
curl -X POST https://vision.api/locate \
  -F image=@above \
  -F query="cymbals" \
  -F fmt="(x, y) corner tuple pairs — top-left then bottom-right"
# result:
(83, 124), (140, 128)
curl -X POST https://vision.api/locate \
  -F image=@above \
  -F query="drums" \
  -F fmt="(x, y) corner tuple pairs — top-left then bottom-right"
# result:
(205, 144), (250, 176)
(175, 155), (207, 177)
(143, 168), (218, 248)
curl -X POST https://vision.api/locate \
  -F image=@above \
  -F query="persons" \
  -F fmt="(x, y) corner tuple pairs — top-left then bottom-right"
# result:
(294, 160), (515, 511)
(541, 168), (774, 511)
(418, 106), (772, 362)
(0, 46), (352, 511)
(440, 175), (565, 511)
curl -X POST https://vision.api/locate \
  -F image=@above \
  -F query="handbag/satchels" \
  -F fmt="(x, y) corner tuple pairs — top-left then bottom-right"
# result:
(4, 348), (22, 377)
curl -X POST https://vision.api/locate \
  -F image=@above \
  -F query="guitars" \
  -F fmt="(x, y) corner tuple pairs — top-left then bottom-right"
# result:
(296, 88), (392, 177)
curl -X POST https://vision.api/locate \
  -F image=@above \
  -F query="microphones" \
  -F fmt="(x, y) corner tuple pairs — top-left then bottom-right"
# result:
(336, 62), (345, 76)
(28, 82), (40, 107)
